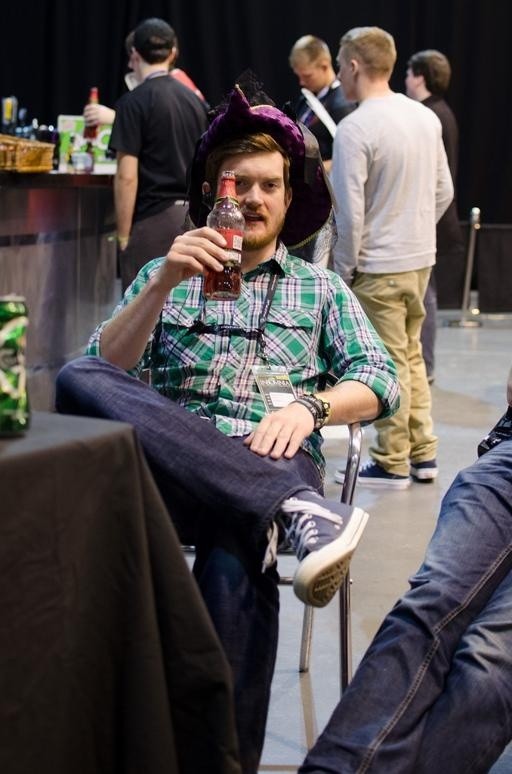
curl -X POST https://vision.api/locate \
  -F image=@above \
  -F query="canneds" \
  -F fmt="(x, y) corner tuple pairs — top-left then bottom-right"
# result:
(2, 296), (29, 434)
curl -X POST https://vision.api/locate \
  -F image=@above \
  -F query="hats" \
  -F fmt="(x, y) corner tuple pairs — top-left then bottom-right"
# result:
(187, 104), (334, 251)
(133, 17), (178, 51)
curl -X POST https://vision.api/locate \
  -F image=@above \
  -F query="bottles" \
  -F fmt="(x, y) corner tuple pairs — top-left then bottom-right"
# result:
(206, 170), (244, 300)
(83, 142), (94, 174)
(83, 86), (99, 138)
(3, 96), (58, 167)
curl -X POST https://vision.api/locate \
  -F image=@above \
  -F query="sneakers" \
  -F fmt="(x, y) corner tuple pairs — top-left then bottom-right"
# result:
(274, 488), (370, 608)
(410, 458), (439, 480)
(334, 460), (413, 490)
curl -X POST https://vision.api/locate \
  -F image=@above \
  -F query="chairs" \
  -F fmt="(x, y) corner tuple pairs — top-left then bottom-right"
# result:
(179, 423), (363, 753)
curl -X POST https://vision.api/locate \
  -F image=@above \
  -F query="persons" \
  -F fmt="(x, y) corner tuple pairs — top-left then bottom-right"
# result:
(406, 50), (462, 382)
(296, 368), (512, 773)
(109, 18), (208, 298)
(281, 35), (358, 264)
(337, 25), (455, 487)
(84, 30), (204, 125)
(56, 84), (401, 773)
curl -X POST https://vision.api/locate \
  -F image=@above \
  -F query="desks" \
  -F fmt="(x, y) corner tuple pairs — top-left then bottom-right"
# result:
(0, 415), (239, 774)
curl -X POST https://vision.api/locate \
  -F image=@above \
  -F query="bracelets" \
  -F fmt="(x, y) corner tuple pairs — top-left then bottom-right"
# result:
(292, 393), (330, 433)
(106, 235), (133, 246)
